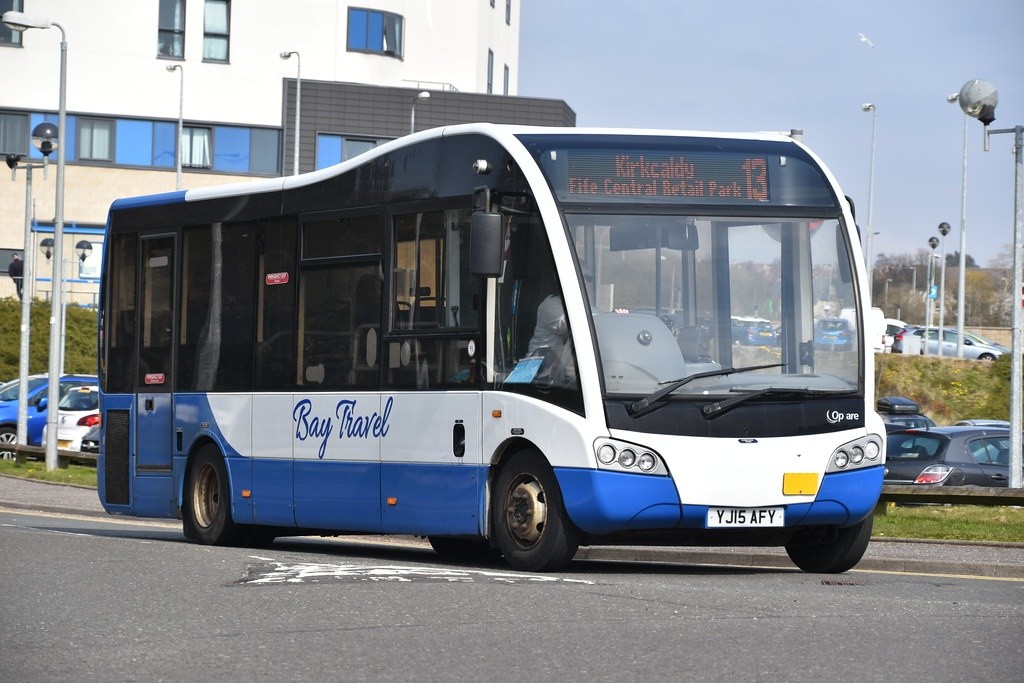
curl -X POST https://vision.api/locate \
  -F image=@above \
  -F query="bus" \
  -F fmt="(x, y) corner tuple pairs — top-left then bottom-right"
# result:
(96, 121), (888, 575)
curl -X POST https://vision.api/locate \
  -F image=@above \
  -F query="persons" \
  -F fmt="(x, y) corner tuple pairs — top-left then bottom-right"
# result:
(333, 274), (398, 367)
(528, 260), (629, 389)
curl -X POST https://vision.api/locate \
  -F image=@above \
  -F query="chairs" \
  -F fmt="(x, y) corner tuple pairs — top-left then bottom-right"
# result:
(59, 385), (66, 402)
(997, 448), (1009, 465)
(912, 445), (929, 459)
(397, 301), (411, 323)
(75, 399), (93, 409)
(420, 297), (446, 329)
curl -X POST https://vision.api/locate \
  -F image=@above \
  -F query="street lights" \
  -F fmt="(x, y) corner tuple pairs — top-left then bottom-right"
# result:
(947, 93), (969, 360)
(959, 79), (1023, 488)
(3, 11), (68, 472)
(410, 91), (430, 134)
(167, 65), (184, 190)
(40, 238), (93, 373)
(280, 51), (300, 175)
(6, 122), (59, 445)
(862, 103), (893, 317)
(909, 221), (950, 356)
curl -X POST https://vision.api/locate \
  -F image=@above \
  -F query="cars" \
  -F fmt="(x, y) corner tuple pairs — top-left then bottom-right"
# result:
(954, 420), (1010, 428)
(629, 307), (713, 340)
(892, 325), (1012, 361)
(0, 373), (98, 461)
(731, 316), (854, 351)
(875, 396), (938, 428)
(41, 386), (101, 451)
(878, 426), (1023, 506)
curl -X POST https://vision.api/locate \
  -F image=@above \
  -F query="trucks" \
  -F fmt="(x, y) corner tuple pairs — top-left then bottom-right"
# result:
(839, 307), (908, 353)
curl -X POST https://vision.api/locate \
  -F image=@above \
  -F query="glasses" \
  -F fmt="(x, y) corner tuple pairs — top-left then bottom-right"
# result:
(583, 274), (594, 283)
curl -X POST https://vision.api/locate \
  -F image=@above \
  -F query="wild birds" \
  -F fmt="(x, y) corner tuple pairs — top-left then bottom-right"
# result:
(859, 32), (874, 48)
